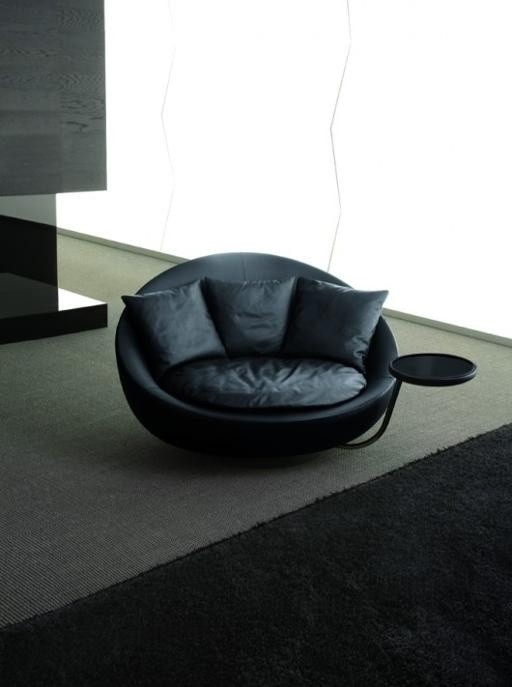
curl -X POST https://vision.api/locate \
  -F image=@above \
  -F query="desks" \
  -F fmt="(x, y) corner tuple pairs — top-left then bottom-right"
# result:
(336, 352), (478, 450)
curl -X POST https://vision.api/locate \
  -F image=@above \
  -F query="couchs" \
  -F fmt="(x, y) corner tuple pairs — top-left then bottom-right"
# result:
(114, 251), (398, 469)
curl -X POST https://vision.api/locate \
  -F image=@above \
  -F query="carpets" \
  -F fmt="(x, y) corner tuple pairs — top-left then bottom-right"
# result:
(2, 424), (510, 684)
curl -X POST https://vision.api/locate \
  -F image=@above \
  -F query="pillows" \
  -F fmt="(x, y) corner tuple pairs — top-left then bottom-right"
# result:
(120, 276), (390, 374)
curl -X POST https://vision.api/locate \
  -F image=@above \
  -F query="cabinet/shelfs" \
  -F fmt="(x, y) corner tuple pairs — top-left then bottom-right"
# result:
(0, 1), (110, 343)
